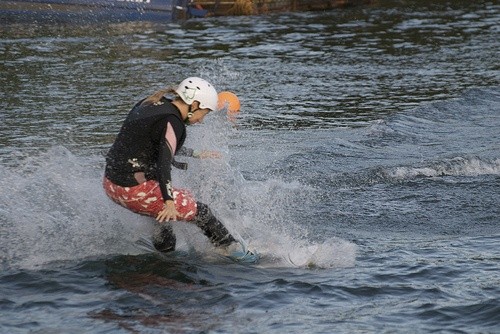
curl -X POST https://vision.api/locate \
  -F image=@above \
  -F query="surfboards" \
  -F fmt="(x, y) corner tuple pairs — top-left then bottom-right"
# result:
(193, 244), (318, 267)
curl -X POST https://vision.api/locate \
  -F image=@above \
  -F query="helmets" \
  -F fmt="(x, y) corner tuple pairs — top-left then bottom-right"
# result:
(174, 77), (218, 111)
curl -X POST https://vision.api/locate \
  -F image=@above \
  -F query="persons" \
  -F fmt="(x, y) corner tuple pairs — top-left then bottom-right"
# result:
(102, 76), (264, 258)
